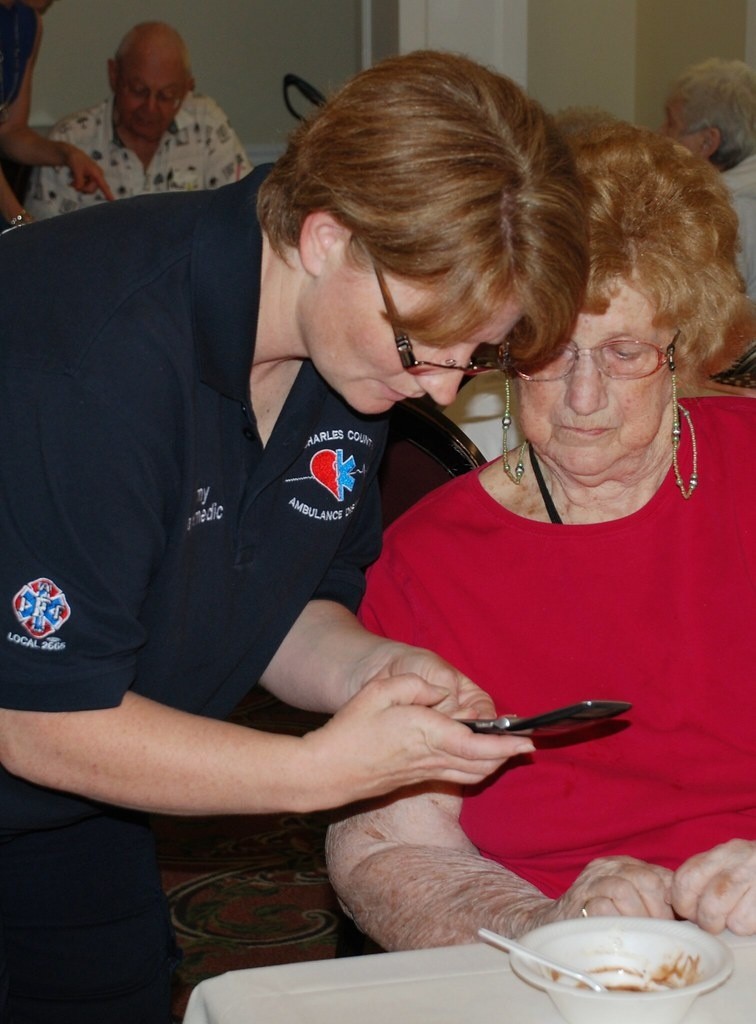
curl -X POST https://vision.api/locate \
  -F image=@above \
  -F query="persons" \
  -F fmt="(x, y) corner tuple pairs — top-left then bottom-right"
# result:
(0, 49), (592, 1024)
(0, 0), (113, 226)
(663, 58), (756, 298)
(25, 21), (253, 222)
(328, 113), (756, 951)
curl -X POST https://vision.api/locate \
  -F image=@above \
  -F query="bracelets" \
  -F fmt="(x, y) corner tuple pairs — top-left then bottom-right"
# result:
(10, 210), (26, 225)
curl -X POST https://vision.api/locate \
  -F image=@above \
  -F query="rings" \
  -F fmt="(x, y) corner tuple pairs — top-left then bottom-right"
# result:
(582, 901), (588, 917)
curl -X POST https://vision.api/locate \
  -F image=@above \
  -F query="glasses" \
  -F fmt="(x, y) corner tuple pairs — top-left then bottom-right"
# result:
(500, 330), (699, 499)
(373, 261), (508, 384)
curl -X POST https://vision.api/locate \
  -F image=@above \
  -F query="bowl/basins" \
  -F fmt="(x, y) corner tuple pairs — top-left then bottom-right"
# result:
(510, 916), (736, 1024)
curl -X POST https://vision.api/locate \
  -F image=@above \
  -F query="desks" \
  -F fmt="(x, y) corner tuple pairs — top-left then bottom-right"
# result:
(184, 921), (756, 1024)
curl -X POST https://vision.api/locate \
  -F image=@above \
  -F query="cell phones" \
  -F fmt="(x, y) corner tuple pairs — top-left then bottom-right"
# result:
(460, 699), (632, 733)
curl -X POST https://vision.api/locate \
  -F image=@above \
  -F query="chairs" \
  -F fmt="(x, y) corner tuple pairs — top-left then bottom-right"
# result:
(162, 389), (488, 1007)
